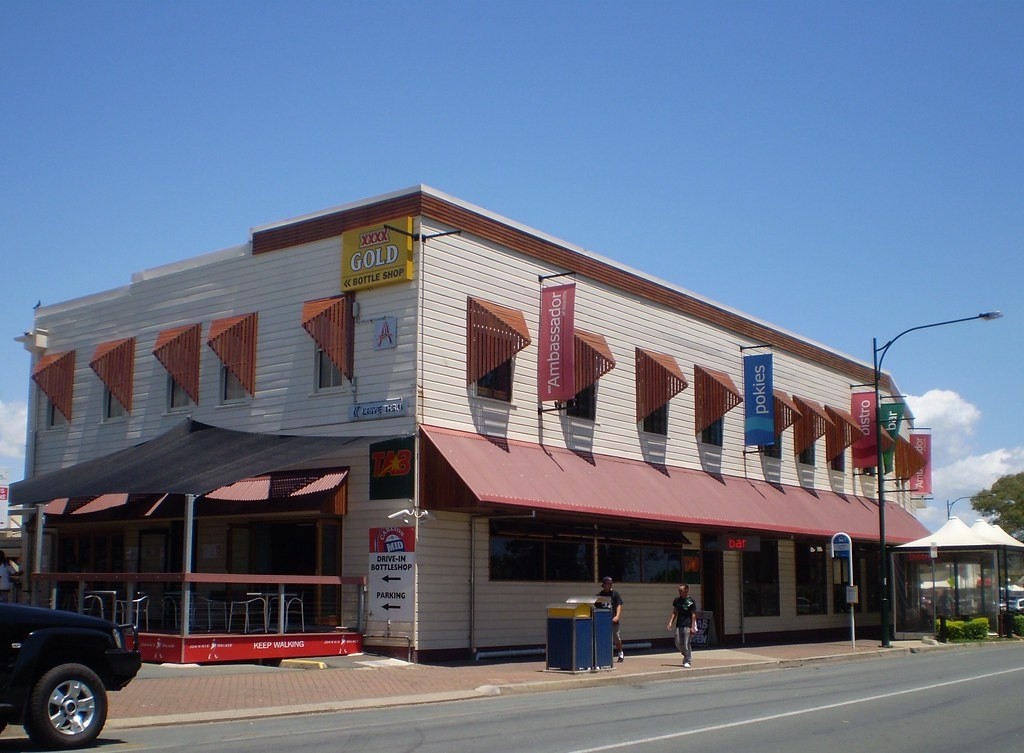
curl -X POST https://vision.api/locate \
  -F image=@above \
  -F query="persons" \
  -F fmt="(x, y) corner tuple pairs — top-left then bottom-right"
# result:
(936, 588), (953, 617)
(668, 584), (697, 667)
(0, 550), (15, 602)
(596, 577), (624, 663)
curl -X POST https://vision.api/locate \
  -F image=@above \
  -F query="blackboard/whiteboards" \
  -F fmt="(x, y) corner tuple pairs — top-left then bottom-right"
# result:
(690, 611), (718, 646)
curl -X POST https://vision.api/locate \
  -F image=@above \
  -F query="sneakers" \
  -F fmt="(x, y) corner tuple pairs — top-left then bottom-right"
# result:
(682, 656), (687, 664)
(684, 663), (691, 668)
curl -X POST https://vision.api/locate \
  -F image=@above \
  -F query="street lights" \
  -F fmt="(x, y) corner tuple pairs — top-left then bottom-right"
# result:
(947, 494), (1016, 620)
(873, 310), (1006, 650)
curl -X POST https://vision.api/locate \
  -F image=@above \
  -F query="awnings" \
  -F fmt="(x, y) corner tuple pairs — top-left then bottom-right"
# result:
(43, 466), (347, 526)
(420, 425), (932, 545)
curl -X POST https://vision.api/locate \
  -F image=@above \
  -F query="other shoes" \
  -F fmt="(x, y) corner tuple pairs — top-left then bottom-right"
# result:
(618, 651), (625, 662)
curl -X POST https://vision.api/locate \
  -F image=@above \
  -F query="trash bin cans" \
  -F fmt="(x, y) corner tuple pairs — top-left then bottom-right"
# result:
(545, 603), (593, 671)
(566, 595), (613, 670)
(1002, 613), (1013, 635)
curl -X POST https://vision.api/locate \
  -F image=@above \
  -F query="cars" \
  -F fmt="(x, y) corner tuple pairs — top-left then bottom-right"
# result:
(0, 602), (143, 752)
(992, 597), (1024, 616)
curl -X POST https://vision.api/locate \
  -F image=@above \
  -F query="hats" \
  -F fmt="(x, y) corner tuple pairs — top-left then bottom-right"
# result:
(602, 577), (614, 584)
(944, 588), (952, 591)
